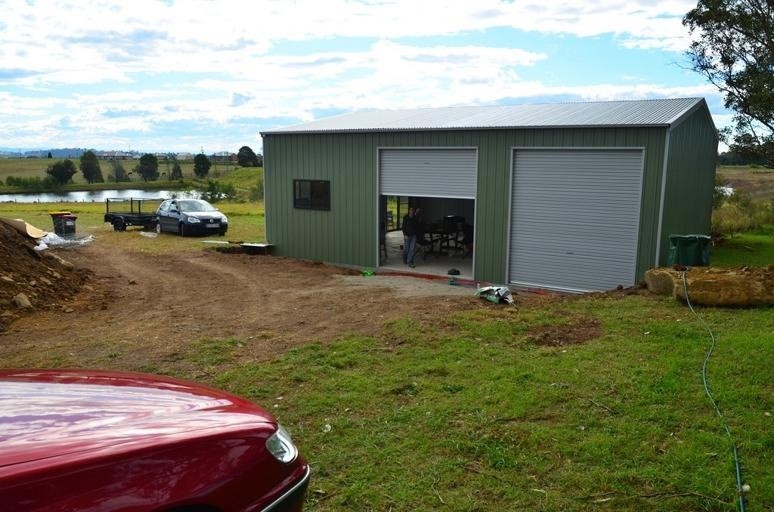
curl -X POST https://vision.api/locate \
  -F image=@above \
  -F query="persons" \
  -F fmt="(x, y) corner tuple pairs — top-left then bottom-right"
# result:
(402, 206), (474, 268)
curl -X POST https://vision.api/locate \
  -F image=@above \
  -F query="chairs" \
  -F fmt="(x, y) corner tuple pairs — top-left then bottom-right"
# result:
(416, 215), (466, 263)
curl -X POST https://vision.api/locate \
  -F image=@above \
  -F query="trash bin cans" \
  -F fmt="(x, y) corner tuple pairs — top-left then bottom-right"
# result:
(671, 235), (712, 266)
(50, 213), (77, 235)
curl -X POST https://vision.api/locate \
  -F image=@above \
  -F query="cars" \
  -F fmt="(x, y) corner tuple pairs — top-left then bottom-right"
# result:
(155, 200), (229, 237)
(1, 367), (312, 511)
